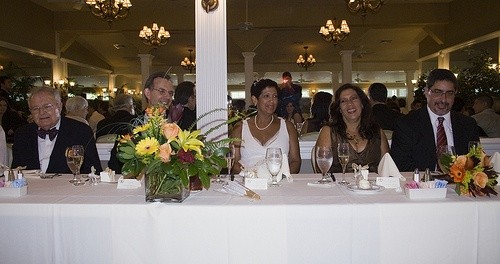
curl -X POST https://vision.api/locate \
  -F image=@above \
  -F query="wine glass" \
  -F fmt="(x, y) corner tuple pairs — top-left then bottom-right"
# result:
(66, 145), (86, 186)
(222, 145), (236, 184)
(337, 142), (351, 186)
(266, 148), (283, 186)
(315, 146), (334, 183)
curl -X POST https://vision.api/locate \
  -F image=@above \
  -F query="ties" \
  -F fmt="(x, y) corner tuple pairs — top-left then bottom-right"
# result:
(436, 117), (448, 173)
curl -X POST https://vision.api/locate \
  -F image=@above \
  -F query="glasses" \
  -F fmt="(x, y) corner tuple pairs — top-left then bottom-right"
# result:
(30, 101), (59, 114)
(427, 86), (457, 98)
(152, 87), (173, 98)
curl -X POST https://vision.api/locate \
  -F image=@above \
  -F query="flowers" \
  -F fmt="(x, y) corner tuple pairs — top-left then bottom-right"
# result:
(112, 108), (245, 175)
(422, 143), (499, 199)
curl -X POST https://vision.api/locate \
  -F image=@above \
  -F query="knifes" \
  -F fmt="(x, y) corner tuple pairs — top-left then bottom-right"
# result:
(330, 172), (337, 183)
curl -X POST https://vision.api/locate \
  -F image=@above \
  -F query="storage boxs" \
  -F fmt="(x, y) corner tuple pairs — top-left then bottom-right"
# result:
(400, 180), (448, 201)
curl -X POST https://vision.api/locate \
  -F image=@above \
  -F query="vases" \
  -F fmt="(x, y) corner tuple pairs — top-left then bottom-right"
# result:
(145, 163), (193, 203)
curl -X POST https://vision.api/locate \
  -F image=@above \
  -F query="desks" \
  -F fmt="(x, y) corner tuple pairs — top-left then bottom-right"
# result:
(0, 173), (500, 264)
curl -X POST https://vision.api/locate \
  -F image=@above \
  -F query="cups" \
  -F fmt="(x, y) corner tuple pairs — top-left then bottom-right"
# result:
(436, 145), (457, 175)
(468, 140), (480, 152)
(296, 122), (303, 135)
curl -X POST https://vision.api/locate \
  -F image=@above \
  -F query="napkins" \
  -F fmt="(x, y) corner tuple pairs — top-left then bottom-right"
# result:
(378, 153), (401, 178)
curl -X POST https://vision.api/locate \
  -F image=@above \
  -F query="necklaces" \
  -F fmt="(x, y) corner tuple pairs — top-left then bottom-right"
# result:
(254, 115), (273, 130)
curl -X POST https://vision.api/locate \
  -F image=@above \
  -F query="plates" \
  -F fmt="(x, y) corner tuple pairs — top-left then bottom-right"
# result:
(348, 184), (385, 193)
(17, 169), (42, 175)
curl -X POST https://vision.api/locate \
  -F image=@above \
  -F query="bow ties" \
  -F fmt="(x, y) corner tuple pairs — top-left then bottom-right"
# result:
(37, 127), (59, 141)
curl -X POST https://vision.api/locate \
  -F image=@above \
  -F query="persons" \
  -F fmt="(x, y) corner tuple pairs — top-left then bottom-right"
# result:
(0, 76), (29, 145)
(231, 79), (302, 175)
(168, 81), (197, 132)
(391, 69), (487, 172)
(227, 95), (258, 132)
(276, 72), (333, 135)
(63, 94), (144, 141)
(367, 83), (422, 131)
(10, 87), (103, 175)
(464, 94), (500, 138)
(315, 83), (390, 174)
(107, 72), (173, 174)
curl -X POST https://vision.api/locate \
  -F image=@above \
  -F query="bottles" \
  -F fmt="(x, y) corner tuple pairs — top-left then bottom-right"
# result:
(7, 169), (24, 181)
(412, 167), (431, 182)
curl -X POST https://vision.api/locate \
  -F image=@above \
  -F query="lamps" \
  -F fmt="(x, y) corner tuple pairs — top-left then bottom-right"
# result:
(315, 15), (350, 48)
(180, 49), (196, 73)
(297, 46), (317, 73)
(138, 21), (171, 49)
(45, 79), (75, 90)
(346, 0), (386, 21)
(83, 0), (131, 20)
